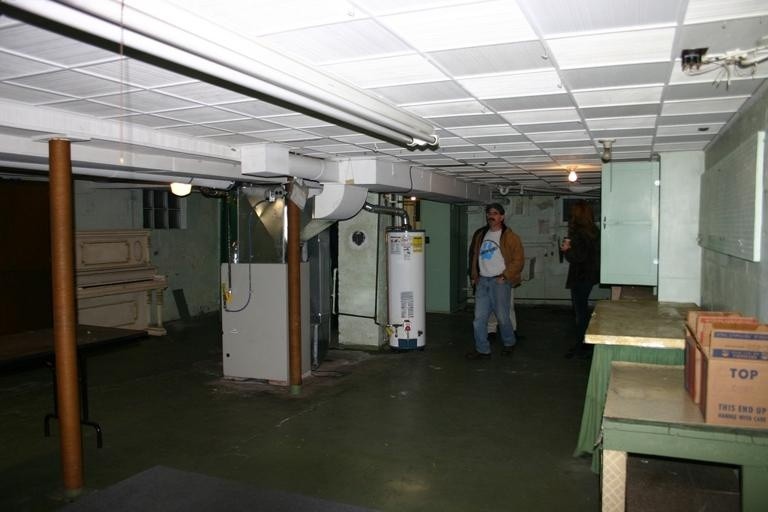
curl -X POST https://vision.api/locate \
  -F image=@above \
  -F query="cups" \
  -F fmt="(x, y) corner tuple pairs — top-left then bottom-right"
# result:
(563, 236), (571, 247)
(233, 246), (239, 266)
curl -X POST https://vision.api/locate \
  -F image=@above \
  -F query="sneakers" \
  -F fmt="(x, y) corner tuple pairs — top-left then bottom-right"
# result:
(503, 346), (513, 353)
(466, 352), (492, 360)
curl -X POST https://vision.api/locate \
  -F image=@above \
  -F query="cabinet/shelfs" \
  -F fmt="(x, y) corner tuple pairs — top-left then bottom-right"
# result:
(600, 160), (657, 288)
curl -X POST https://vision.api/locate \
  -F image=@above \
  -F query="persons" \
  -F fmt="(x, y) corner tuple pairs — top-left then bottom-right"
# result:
(466, 202), (525, 360)
(559, 201), (601, 354)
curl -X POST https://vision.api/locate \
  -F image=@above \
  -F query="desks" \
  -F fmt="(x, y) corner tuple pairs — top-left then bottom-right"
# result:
(0, 323), (148, 449)
(571, 300), (691, 459)
(594, 358), (767, 512)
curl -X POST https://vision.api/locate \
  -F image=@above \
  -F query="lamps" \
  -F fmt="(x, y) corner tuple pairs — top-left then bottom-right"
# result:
(567, 166), (579, 184)
(168, 178), (192, 199)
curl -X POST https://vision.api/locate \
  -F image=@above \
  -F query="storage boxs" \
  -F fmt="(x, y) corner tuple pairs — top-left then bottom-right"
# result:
(683, 306), (767, 430)
